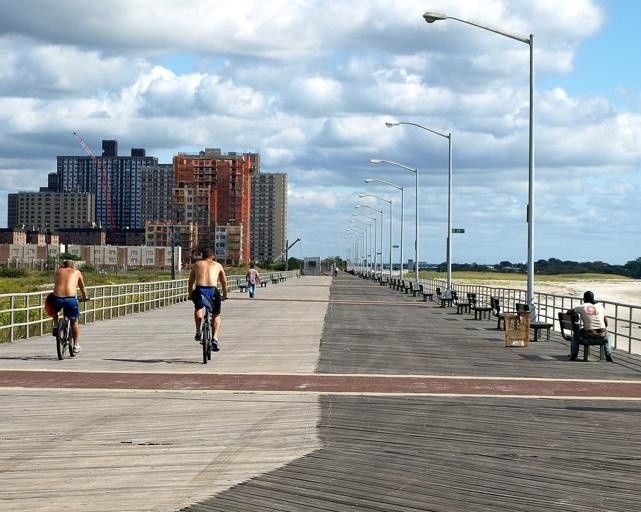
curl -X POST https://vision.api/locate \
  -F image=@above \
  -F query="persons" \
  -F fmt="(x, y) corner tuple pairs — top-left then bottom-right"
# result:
(47, 261), (88, 352)
(245, 263), (260, 299)
(335, 268), (337, 277)
(188, 250), (227, 352)
(566, 291), (614, 362)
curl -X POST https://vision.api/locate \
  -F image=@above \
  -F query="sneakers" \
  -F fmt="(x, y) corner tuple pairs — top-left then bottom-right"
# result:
(212, 337), (220, 351)
(194, 332), (202, 341)
(52, 322), (58, 336)
(72, 346), (81, 356)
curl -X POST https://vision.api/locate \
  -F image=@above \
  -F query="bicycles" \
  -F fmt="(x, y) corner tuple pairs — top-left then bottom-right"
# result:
(188, 297), (228, 363)
(52, 297), (90, 360)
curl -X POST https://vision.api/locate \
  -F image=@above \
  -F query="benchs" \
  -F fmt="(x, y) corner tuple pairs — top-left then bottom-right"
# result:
(346, 266), (610, 366)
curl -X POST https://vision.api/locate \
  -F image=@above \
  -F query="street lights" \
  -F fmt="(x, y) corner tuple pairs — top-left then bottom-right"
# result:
(344, 205), (384, 276)
(365, 177), (406, 282)
(359, 194), (394, 283)
(369, 159), (420, 286)
(422, 11), (537, 317)
(385, 120), (454, 289)
